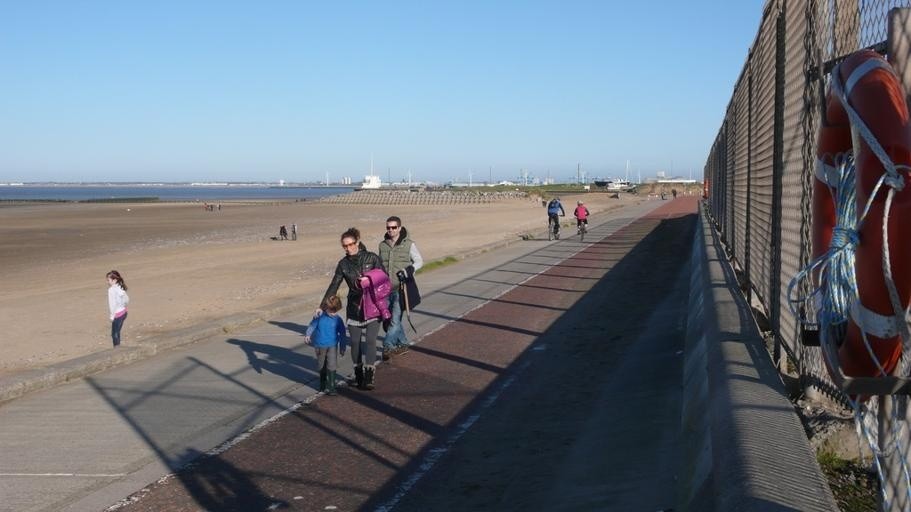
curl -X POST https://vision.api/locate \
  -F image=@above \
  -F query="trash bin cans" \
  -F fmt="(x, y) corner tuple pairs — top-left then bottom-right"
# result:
(662, 192), (667, 200)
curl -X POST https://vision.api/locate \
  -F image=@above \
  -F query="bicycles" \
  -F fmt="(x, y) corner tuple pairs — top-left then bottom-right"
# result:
(576, 220), (588, 241)
(549, 215), (565, 242)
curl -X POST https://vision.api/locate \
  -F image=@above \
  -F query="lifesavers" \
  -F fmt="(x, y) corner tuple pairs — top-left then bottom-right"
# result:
(812, 51), (910, 395)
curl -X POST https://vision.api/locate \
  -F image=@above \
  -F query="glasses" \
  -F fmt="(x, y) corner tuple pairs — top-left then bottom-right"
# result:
(342, 241), (357, 248)
(385, 224), (399, 229)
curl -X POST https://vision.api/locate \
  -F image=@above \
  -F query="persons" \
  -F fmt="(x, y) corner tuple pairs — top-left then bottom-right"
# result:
(305, 296), (347, 396)
(314, 226), (389, 390)
(280, 224), (289, 240)
(574, 200), (590, 235)
(375, 216), (423, 359)
(203, 201), (225, 212)
(291, 223), (297, 240)
(671, 188), (676, 201)
(106, 270), (130, 347)
(548, 196), (565, 237)
(661, 190), (665, 200)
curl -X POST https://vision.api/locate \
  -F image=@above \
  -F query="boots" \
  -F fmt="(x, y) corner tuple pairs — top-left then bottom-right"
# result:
(329, 369), (338, 396)
(364, 365), (376, 390)
(318, 369), (329, 394)
(350, 362), (363, 388)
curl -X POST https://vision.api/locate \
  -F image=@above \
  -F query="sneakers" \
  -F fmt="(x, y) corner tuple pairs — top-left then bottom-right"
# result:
(382, 346), (392, 363)
(393, 344), (411, 355)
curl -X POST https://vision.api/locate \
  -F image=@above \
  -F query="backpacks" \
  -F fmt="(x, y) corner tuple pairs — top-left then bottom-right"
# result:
(550, 198), (556, 209)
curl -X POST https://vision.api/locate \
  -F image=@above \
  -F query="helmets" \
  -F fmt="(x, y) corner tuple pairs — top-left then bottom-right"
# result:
(576, 199), (584, 205)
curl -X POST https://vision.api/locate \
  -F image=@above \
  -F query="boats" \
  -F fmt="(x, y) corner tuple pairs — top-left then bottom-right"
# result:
(594, 181), (630, 188)
